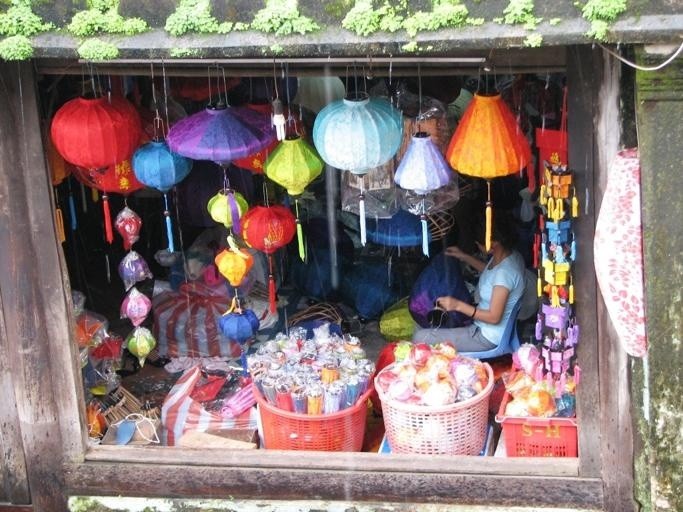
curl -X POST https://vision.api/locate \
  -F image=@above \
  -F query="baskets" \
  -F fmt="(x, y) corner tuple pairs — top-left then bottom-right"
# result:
(252, 381), (374, 451)
(374, 358), (494, 456)
(495, 365), (578, 458)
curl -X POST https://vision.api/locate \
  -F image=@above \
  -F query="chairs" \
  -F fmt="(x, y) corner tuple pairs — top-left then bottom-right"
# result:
(452, 279), (528, 362)
